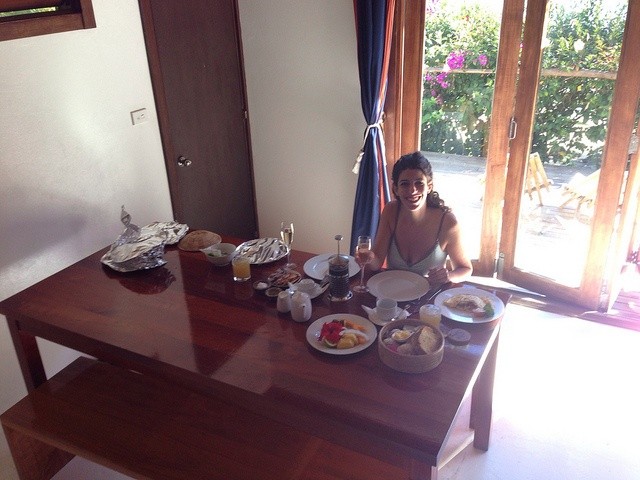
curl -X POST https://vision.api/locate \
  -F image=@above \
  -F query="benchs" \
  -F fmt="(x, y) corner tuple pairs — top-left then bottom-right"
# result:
(1, 357), (420, 479)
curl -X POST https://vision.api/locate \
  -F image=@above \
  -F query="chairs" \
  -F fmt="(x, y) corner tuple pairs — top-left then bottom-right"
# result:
(557, 168), (628, 217)
(523, 153), (555, 207)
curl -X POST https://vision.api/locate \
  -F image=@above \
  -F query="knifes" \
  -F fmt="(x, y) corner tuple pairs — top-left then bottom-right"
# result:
(413, 288), (443, 313)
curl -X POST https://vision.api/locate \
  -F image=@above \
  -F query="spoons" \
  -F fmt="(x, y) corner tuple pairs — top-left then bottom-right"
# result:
(388, 304), (411, 322)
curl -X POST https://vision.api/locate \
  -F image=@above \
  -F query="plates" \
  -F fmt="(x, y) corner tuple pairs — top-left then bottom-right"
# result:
(366, 269), (431, 302)
(177, 226), (223, 254)
(303, 252), (362, 281)
(435, 286), (505, 324)
(306, 313), (378, 356)
(287, 284), (325, 299)
(236, 238), (289, 265)
(367, 307), (410, 326)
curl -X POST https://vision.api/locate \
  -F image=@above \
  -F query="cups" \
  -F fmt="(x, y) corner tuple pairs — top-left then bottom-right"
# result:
(276, 289), (292, 314)
(232, 254), (252, 282)
(376, 299), (399, 318)
(419, 304), (440, 331)
(296, 279), (316, 292)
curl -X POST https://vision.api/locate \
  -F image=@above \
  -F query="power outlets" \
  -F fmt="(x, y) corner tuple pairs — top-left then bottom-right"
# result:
(131, 107), (148, 125)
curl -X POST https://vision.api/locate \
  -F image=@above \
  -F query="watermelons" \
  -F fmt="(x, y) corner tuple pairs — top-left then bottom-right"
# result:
(319, 320), (343, 349)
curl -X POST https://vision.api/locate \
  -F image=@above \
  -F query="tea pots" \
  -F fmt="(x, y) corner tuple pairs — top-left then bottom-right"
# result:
(290, 291), (313, 323)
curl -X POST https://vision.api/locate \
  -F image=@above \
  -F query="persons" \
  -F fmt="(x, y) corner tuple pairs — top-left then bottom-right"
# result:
(355, 151), (473, 285)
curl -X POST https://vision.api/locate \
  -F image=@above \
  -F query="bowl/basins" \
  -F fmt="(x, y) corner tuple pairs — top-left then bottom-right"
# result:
(252, 279), (271, 290)
(265, 287), (283, 299)
(377, 318), (454, 362)
(204, 242), (236, 268)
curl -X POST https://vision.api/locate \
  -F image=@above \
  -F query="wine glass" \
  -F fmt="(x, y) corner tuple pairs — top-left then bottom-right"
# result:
(280, 222), (297, 272)
(353, 234), (372, 294)
